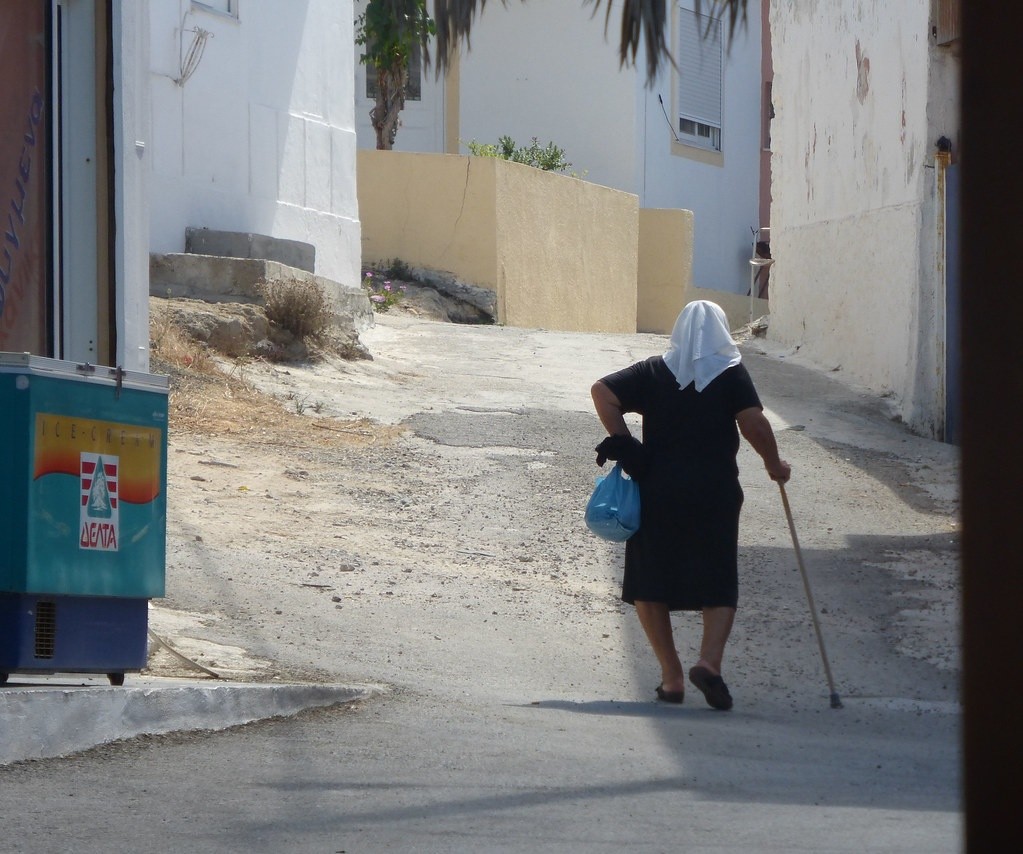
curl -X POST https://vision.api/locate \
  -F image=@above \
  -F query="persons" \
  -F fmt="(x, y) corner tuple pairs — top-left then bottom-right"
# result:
(582, 298), (794, 713)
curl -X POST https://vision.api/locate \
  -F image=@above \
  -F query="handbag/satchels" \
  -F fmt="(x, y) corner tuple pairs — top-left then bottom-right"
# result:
(583, 465), (639, 542)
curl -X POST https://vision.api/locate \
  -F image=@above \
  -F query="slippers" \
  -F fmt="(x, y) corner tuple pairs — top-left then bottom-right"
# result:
(688, 666), (732, 710)
(655, 682), (684, 703)
